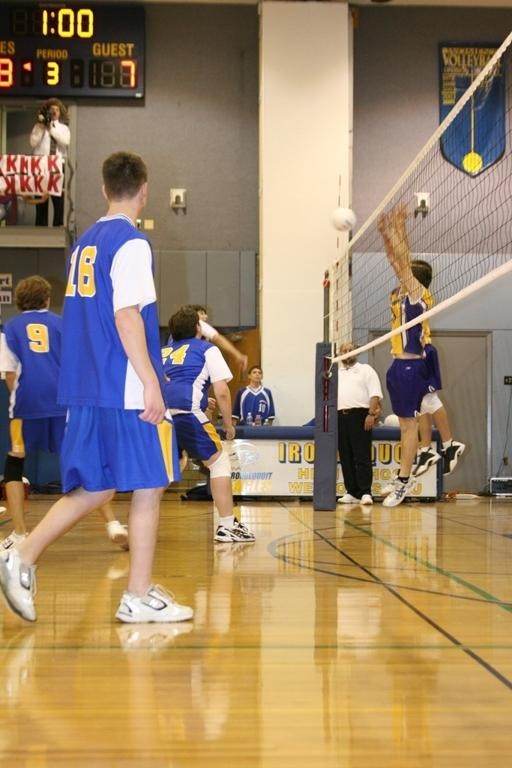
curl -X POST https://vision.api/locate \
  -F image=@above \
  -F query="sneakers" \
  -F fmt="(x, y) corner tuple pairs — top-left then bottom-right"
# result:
(1, 536), (38, 623)
(214, 517), (256, 542)
(106, 520), (129, 550)
(337, 440), (465, 507)
(116, 586), (193, 622)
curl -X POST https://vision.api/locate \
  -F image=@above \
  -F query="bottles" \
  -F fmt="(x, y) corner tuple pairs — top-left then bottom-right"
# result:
(254, 414), (263, 427)
(245, 411), (253, 426)
(217, 412), (223, 425)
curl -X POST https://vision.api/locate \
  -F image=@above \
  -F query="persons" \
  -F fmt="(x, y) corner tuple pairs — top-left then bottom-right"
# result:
(160, 304), (256, 543)
(1, 276), (129, 554)
(30, 98), (71, 227)
(165, 304), (248, 497)
(338, 345), (383, 505)
(0, 151), (193, 622)
(231, 365), (275, 426)
(377, 201), (465, 507)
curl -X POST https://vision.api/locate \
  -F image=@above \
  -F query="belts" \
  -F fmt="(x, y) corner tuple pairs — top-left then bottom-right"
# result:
(338, 409), (368, 415)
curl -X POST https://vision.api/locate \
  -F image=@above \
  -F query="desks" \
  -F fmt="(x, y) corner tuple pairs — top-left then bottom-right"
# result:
(211, 422), (445, 501)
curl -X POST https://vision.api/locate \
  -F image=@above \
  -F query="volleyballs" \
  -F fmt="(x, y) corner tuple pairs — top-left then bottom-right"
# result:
(332, 206), (355, 229)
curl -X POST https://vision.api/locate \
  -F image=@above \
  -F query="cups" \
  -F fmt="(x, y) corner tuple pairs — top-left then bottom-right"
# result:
(263, 418), (273, 427)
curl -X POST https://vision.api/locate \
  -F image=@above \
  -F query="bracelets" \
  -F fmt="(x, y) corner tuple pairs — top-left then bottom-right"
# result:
(368, 413), (375, 416)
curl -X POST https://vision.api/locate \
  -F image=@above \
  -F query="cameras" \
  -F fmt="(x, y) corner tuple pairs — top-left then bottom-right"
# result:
(42, 108), (51, 126)
